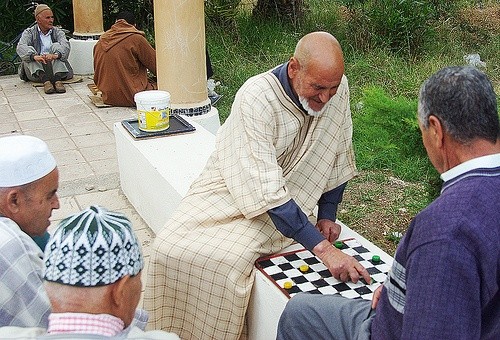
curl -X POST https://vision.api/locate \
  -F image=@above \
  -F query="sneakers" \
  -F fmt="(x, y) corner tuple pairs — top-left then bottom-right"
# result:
(208, 95), (223, 106)
(43, 81), (66, 94)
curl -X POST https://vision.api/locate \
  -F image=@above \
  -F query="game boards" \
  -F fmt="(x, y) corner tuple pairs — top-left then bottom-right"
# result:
(255, 238), (393, 300)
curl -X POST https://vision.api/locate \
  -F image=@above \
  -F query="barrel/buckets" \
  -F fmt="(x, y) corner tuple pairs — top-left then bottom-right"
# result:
(134, 89), (171, 133)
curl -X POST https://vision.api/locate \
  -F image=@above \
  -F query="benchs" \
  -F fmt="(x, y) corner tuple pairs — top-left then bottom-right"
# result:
(115, 115), (394, 340)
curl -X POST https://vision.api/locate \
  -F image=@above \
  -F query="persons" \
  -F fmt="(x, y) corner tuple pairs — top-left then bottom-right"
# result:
(138, 31), (373, 340)
(16, 4), (73, 95)
(92, 10), (224, 107)
(0, 203), (183, 340)
(1, 133), (150, 330)
(275, 66), (500, 340)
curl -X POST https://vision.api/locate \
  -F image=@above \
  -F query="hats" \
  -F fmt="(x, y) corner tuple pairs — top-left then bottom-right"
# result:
(41, 204), (145, 287)
(35, 4), (51, 16)
(0, 135), (57, 187)
